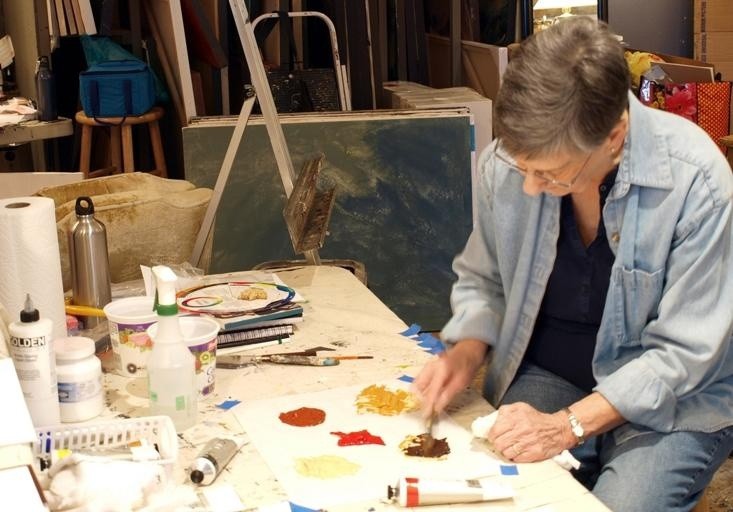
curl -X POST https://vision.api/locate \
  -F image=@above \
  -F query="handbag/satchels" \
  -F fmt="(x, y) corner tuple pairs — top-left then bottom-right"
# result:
(638, 73), (733, 163)
(79, 59), (157, 127)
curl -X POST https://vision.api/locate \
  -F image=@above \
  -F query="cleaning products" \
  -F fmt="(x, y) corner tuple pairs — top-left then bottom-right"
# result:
(146, 265), (195, 424)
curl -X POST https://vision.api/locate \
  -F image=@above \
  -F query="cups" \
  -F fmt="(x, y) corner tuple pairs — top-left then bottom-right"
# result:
(146, 316), (222, 399)
(103, 294), (159, 379)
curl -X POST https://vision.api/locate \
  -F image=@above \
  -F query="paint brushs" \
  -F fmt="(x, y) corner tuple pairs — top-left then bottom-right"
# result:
(215, 350), (374, 366)
(424, 415), (437, 452)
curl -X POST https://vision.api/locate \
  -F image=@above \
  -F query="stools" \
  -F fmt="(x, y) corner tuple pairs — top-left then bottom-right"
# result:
(75, 106), (168, 178)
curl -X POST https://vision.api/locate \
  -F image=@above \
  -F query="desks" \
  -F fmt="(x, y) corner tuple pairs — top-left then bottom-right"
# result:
(0, 116), (75, 172)
(0, 266), (610, 512)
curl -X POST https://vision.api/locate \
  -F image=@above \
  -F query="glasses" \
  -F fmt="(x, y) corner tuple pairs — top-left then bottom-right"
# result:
(492, 138), (598, 194)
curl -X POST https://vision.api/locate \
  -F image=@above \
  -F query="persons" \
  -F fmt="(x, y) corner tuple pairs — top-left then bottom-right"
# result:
(411, 15), (732, 512)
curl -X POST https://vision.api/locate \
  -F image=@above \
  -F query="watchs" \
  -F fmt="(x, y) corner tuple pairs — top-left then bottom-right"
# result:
(561, 406), (585, 447)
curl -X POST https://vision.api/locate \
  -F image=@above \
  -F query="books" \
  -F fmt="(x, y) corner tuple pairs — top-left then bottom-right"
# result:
(177, 269), (304, 357)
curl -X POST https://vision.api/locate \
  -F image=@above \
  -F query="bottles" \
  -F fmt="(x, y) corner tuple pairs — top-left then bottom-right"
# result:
(9, 294), (61, 428)
(66, 196), (113, 328)
(52, 335), (108, 423)
